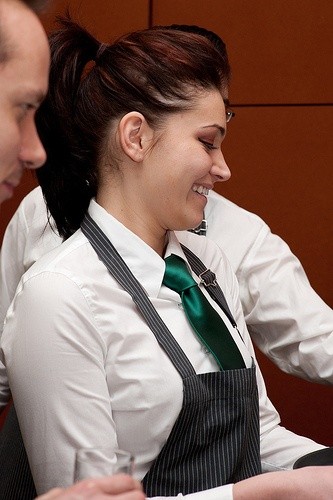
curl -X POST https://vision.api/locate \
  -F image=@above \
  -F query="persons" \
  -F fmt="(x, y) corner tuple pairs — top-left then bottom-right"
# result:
(1, 13), (332, 499)
(1, 1), (144, 500)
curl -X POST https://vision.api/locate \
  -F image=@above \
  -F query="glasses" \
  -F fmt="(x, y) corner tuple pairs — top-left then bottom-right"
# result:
(226, 111), (236, 122)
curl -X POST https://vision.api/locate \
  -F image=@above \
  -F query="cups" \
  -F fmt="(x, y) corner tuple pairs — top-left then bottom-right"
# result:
(74, 448), (135, 487)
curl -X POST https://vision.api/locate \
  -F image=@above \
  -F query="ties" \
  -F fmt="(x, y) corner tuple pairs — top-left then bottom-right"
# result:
(163, 255), (245, 370)
